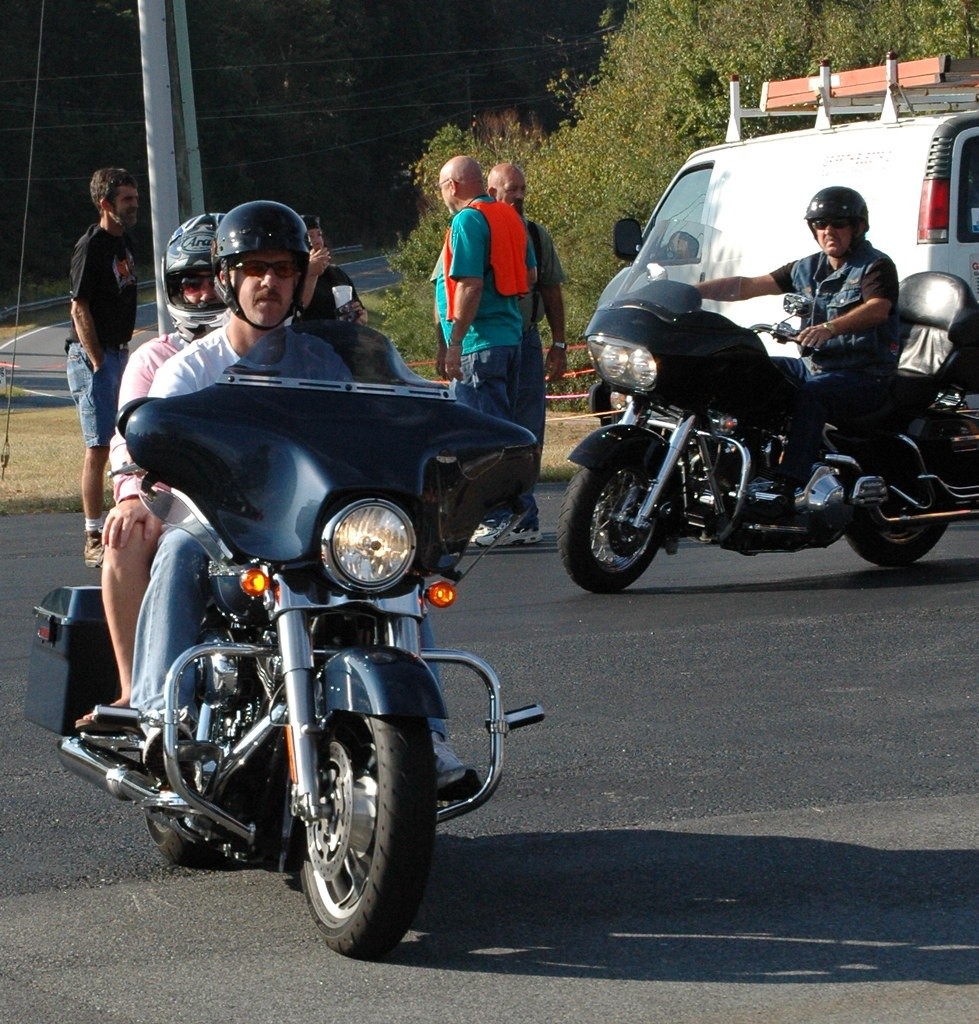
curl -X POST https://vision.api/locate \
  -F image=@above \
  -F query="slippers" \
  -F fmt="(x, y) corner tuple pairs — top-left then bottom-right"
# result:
(76, 713), (138, 737)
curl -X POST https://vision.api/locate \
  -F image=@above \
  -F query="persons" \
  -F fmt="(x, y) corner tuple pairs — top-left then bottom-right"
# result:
(65, 168), (138, 568)
(690, 186), (898, 512)
(72, 214), (230, 727)
(126, 200), (481, 801)
(430, 155), (569, 546)
(297, 215), (369, 326)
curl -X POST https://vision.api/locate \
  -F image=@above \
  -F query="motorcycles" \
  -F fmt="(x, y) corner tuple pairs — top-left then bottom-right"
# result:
(558, 219), (979, 594)
(24, 319), (547, 959)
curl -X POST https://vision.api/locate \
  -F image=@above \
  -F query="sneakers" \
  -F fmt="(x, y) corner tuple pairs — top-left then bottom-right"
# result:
(476, 518), (542, 547)
(144, 726), (196, 793)
(468, 523), (497, 545)
(434, 734), (481, 802)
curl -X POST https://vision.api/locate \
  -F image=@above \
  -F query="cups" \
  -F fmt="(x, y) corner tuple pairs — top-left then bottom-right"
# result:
(332, 285), (352, 309)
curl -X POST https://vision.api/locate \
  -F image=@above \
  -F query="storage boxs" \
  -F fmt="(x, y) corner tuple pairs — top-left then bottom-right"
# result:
(906, 413), (979, 490)
(23, 585), (119, 736)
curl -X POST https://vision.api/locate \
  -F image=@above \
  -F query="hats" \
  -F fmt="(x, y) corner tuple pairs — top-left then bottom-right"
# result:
(301, 214), (320, 230)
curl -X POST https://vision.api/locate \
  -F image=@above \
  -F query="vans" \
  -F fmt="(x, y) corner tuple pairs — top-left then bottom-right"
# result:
(596, 109), (979, 324)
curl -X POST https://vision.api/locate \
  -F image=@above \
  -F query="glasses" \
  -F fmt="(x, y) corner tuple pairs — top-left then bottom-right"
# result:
(810, 219), (855, 231)
(230, 260), (299, 277)
(179, 277), (217, 294)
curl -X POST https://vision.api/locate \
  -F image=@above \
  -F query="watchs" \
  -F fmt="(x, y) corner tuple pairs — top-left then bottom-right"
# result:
(822, 321), (836, 336)
(553, 342), (567, 351)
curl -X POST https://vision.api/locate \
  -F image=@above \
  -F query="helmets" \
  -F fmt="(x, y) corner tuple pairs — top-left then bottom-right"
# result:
(212, 200), (310, 287)
(163, 214), (230, 341)
(805, 188), (869, 243)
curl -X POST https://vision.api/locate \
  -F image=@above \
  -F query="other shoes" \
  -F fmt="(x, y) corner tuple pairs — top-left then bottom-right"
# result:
(761, 483), (802, 500)
(82, 532), (103, 568)
